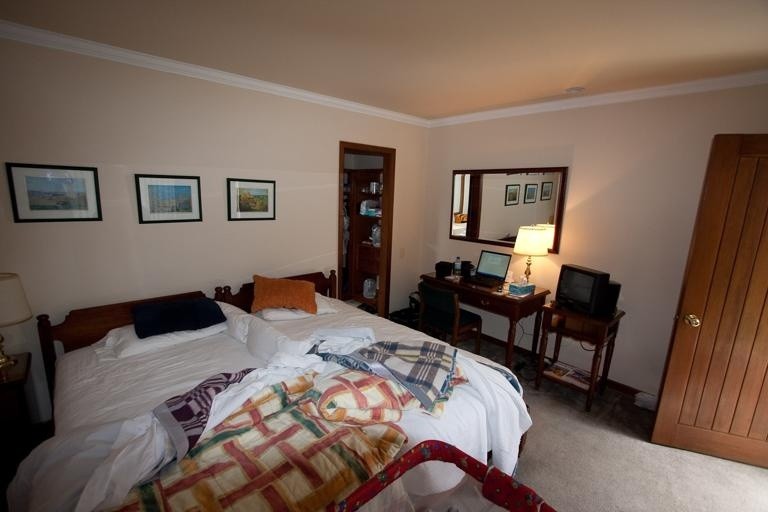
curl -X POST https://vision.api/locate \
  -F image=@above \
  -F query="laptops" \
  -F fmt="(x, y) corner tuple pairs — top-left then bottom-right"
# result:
(461, 249), (512, 288)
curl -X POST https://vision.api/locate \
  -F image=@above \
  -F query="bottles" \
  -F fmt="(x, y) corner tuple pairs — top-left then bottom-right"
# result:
(455, 256), (462, 276)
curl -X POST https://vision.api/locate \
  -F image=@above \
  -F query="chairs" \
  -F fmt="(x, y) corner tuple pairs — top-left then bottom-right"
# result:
(418, 282), (482, 357)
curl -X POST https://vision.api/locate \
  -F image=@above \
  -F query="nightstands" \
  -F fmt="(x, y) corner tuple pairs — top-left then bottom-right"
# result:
(37, 287), (393, 512)
(0, 353), (39, 456)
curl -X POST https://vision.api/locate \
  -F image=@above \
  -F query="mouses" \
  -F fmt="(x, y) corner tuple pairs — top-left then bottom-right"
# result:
(497, 285), (503, 293)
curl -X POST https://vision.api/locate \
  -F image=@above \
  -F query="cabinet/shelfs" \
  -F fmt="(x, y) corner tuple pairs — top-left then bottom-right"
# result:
(348, 171), (384, 306)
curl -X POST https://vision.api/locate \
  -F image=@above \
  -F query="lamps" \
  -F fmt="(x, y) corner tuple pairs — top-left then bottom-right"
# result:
(0, 274), (32, 366)
(513, 226), (548, 283)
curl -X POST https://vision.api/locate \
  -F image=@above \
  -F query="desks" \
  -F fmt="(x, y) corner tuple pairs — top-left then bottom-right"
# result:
(420, 272), (550, 370)
(533, 302), (625, 414)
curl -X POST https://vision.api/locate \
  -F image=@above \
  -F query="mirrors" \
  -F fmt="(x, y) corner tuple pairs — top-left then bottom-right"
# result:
(449, 168), (568, 254)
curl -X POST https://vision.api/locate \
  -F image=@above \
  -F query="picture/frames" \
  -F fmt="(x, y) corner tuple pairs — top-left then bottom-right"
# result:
(505, 185), (520, 206)
(135, 174), (202, 223)
(6, 162), (102, 222)
(541, 182), (553, 201)
(524, 184), (538, 203)
(228, 178), (276, 220)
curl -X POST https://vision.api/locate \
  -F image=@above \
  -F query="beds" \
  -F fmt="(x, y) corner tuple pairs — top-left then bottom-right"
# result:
(223, 269), (521, 501)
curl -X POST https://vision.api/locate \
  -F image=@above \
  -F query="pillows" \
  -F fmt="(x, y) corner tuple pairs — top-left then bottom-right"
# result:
(262, 296), (336, 321)
(132, 295), (224, 338)
(252, 275), (316, 314)
(92, 323), (227, 361)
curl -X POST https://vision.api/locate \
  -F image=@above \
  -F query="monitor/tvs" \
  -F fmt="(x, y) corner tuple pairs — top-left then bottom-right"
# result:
(555, 264), (621, 317)
